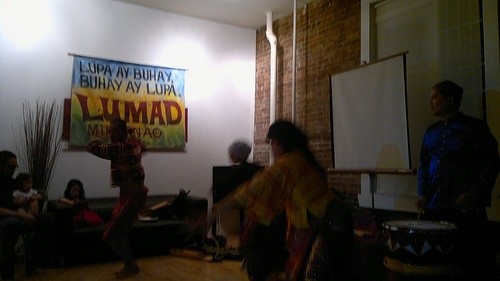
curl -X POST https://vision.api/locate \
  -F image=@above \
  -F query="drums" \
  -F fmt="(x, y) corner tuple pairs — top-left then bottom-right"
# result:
(380, 218), (458, 274)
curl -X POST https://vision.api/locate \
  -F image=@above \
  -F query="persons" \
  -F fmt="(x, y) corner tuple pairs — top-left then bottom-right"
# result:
(87, 118), (146, 277)
(192, 120), (355, 281)
(12, 173), (41, 217)
(0, 151), (40, 281)
(416, 81), (500, 281)
(58, 179), (101, 225)
(229, 142), (251, 166)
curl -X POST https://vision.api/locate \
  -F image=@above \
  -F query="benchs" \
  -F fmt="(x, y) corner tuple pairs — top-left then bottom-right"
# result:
(47, 194), (208, 267)
